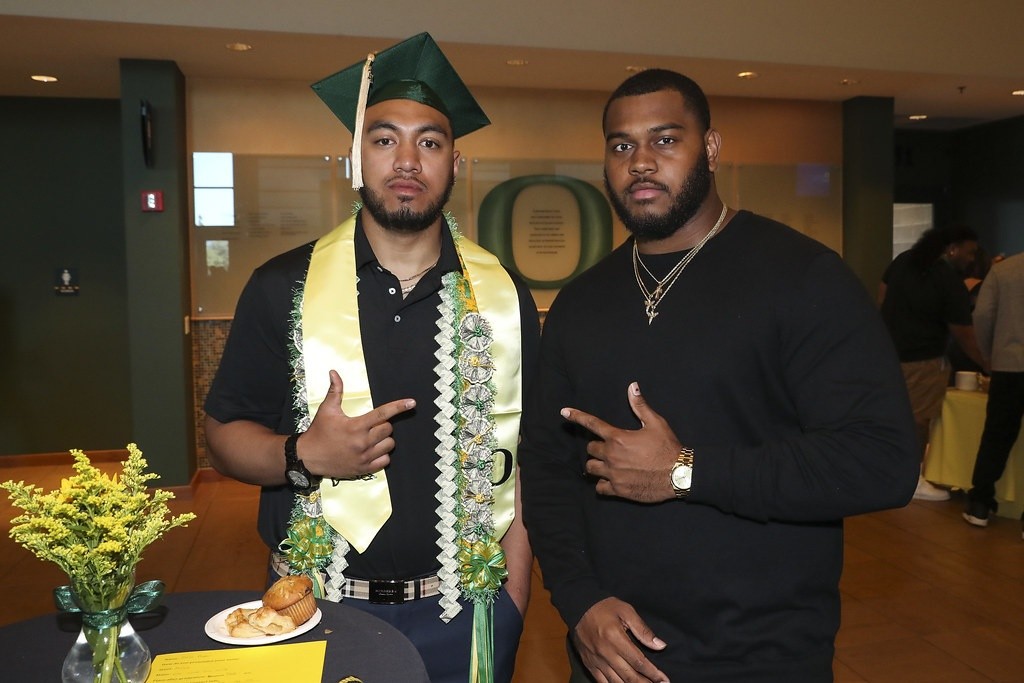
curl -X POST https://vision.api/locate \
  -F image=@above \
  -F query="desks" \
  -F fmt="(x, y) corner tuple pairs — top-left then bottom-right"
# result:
(923, 386), (1024, 520)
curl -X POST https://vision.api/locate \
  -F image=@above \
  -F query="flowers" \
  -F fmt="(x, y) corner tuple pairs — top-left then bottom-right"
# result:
(0, 442), (196, 683)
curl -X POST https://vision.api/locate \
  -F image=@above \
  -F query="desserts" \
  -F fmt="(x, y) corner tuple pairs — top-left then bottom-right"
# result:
(262, 575), (318, 627)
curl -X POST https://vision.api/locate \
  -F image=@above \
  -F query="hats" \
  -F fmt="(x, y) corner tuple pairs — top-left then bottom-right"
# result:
(311, 32), (492, 191)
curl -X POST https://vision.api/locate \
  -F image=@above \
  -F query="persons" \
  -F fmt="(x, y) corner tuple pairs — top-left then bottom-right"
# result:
(201, 34), (543, 683)
(879, 225), (1005, 501)
(515, 67), (920, 683)
(964, 251), (1024, 540)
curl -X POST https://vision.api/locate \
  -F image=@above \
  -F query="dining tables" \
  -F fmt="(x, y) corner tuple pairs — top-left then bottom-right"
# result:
(0, 589), (430, 683)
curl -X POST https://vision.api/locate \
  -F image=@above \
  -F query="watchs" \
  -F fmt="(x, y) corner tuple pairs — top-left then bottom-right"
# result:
(670, 447), (694, 498)
(284, 433), (322, 490)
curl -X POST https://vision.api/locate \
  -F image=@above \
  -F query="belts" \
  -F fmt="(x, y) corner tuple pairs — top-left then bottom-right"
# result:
(271, 549), (441, 604)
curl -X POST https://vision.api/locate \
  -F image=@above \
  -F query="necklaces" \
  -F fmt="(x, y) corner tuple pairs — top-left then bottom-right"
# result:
(399, 262), (437, 294)
(632, 203), (728, 324)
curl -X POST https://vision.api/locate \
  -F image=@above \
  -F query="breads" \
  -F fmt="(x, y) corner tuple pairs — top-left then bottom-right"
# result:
(224, 607), (267, 638)
(248, 606), (296, 635)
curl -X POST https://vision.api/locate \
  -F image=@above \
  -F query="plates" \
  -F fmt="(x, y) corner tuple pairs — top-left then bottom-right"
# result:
(204, 600), (323, 645)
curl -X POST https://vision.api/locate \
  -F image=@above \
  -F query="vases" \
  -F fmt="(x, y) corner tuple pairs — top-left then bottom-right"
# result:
(61, 567), (153, 683)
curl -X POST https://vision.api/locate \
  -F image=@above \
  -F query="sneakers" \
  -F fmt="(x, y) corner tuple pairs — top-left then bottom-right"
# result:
(962, 509), (989, 525)
(912, 475), (952, 500)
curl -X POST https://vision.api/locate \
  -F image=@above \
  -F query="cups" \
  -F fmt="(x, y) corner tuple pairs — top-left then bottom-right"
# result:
(955, 371), (979, 391)
